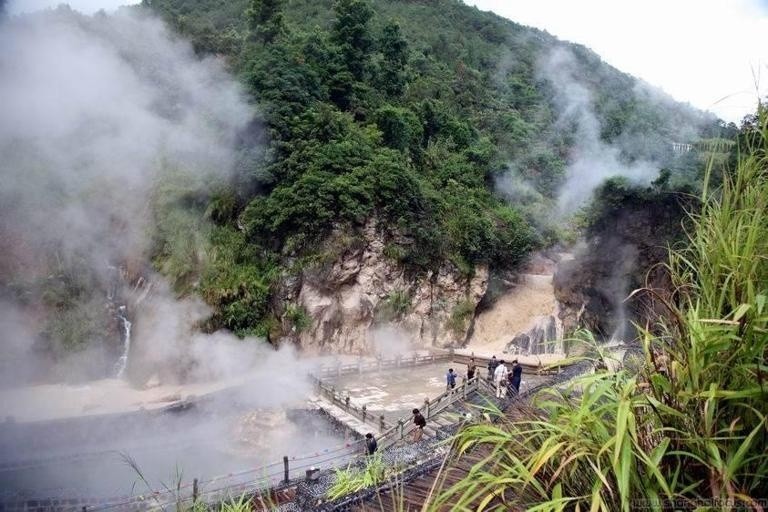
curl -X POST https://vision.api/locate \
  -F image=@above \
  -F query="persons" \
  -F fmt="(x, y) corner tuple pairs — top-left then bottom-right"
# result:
(445, 368), (457, 398)
(467, 357), (476, 384)
(412, 408), (426, 443)
(363, 433), (378, 455)
(488, 355), (523, 400)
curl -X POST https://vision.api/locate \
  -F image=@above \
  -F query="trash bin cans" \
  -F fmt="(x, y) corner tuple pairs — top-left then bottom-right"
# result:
(306, 467), (319, 482)
